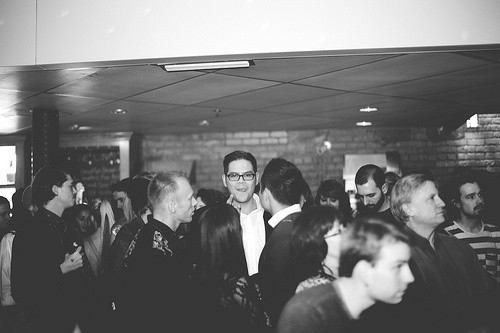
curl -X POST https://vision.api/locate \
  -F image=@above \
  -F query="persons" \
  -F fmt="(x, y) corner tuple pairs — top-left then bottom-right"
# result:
(222, 151), (273, 277)
(0, 158), (500, 333)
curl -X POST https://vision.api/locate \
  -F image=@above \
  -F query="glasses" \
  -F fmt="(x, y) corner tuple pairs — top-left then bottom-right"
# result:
(226, 173), (256, 182)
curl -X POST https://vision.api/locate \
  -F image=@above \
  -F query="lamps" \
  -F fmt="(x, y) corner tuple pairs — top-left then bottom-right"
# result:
(159, 60), (256, 72)
